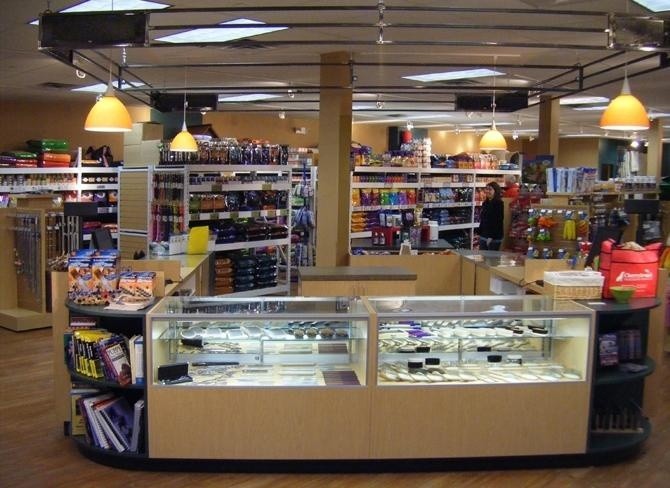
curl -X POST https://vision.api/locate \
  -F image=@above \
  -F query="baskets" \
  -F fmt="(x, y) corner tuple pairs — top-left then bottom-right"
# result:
(542, 269), (605, 302)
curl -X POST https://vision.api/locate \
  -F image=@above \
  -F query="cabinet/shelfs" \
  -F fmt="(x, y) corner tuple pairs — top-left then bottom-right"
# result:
(0, 146), (119, 241)
(62, 294), (663, 471)
(151, 163), (663, 298)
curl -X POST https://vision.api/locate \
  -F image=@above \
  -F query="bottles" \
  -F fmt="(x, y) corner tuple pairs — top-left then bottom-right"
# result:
(374, 232), (385, 246)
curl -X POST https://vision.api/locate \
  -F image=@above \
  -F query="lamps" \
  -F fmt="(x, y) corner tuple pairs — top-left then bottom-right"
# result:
(83, 0), (135, 133)
(169, 59), (199, 154)
(479, 56), (508, 151)
(599, 49), (651, 131)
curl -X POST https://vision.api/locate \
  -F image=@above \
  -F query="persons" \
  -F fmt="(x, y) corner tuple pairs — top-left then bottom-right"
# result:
(476, 180), (506, 265)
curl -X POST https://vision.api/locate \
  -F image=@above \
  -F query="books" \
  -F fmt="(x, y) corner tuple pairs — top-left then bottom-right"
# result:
(60, 317), (147, 455)
(598, 323), (650, 375)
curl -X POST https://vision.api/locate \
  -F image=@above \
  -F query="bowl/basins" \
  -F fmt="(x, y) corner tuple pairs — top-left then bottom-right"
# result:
(610, 287), (635, 303)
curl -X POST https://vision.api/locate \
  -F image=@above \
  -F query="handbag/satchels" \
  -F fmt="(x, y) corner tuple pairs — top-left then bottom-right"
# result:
(86, 144), (113, 167)
